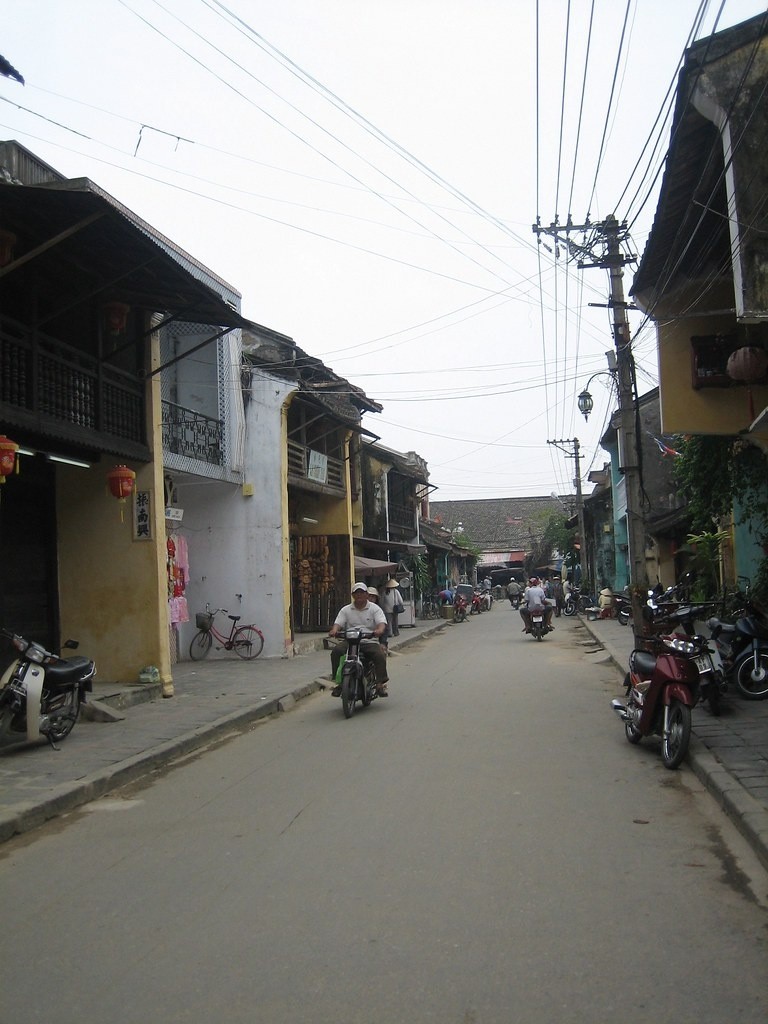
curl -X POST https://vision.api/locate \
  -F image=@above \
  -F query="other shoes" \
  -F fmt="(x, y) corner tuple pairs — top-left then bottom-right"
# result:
(332, 686), (342, 696)
(376, 688), (388, 697)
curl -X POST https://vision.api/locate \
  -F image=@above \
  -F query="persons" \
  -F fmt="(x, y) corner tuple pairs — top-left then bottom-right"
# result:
(519, 577), (554, 632)
(328, 581), (389, 698)
(382, 578), (403, 637)
(484, 576), (492, 594)
(438, 590), (453, 605)
(508, 575), (573, 614)
(598, 588), (613, 610)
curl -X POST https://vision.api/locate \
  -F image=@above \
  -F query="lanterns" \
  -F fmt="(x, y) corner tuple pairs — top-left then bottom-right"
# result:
(0, 435), (19, 484)
(725, 345), (768, 420)
(105, 466), (137, 523)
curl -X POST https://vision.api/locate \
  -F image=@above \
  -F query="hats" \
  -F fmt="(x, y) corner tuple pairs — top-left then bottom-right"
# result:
(352, 582), (367, 592)
(528, 578), (537, 585)
(510, 578), (515, 581)
(367, 587), (379, 596)
(439, 591), (445, 599)
(600, 588), (612, 595)
(385, 579), (399, 587)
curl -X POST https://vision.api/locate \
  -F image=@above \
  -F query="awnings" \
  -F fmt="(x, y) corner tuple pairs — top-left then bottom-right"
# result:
(356, 537), (426, 555)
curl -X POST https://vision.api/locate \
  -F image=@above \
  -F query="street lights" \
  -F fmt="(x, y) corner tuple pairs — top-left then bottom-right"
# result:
(577, 370), (649, 650)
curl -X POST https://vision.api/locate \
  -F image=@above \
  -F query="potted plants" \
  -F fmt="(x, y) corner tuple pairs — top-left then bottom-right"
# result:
(707, 592), (746, 641)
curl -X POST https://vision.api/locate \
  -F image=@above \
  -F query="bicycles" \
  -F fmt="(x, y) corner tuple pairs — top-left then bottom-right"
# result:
(731, 572), (752, 622)
(576, 592), (593, 615)
(422, 588), (441, 620)
(189, 603), (265, 662)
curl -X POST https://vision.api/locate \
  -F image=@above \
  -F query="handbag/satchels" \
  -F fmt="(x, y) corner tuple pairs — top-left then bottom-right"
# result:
(393, 604), (404, 613)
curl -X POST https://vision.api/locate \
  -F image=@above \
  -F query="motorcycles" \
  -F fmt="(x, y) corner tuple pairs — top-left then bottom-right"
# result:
(329, 628), (388, 719)
(511, 595), (520, 610)
(603, 569), (691, 634)
(552, 587), (580, 617)
(0, 627), (98, 751)
(453, 591), (469, 622)
(470, 589), (493, 615)
(706, 589), (768, 701)
(609, 631), (716, 770)
(652, 603), (729, 718)
(519, 593), (553, 642)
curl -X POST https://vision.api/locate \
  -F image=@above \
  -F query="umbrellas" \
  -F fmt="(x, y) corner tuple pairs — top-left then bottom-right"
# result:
(354, 556), (398, 577)
